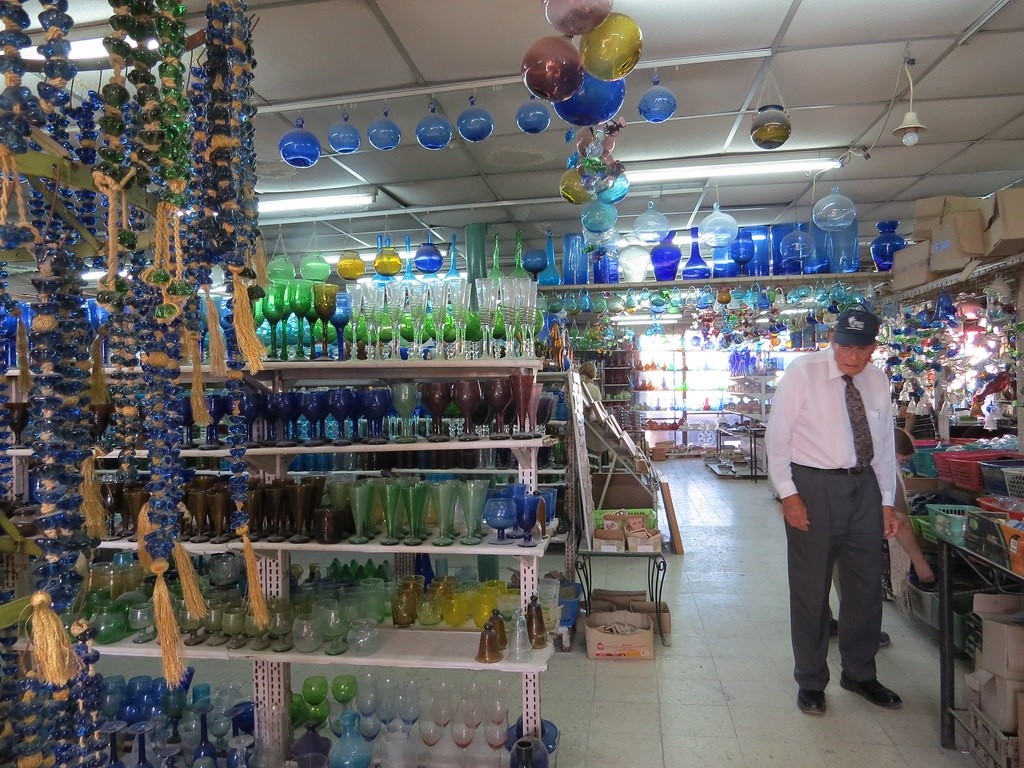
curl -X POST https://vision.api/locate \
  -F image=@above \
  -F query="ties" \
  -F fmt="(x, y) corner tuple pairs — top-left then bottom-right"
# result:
(842, 373), (875, 466)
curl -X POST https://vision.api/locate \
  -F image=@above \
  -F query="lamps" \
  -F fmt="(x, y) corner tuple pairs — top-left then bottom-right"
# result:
(890, 56), (927, 147)
(623, 156), (843, 186)
(617, 229), (691, 248)
(257, 184), (378, 213)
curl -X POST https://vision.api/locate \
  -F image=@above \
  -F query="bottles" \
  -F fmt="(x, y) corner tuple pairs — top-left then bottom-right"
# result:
(375, 219), (907, 285)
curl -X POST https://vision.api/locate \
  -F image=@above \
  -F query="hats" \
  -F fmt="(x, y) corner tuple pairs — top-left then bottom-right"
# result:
(832, 310), (878, 347)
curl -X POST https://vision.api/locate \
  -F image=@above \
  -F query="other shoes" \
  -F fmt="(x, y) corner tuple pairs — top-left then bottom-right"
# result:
(878, 631), (890, 648)
(828, 617), (839, 634)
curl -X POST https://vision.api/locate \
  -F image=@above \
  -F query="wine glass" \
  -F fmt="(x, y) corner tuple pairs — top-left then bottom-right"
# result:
(0, 276), (559, 768)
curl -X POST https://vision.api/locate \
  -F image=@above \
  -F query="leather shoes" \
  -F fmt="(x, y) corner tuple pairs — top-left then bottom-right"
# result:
(839, 669), (904, 706)
(797, 678), (825, 716)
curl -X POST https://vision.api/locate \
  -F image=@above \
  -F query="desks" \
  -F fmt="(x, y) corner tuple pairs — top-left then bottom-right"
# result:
(624, 429), (647, 456)
(574, 537), (672, 647)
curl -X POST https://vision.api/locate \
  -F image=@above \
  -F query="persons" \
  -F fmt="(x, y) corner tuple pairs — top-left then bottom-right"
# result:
(763, 308), (935, 716)
(882, 356), (937, 440)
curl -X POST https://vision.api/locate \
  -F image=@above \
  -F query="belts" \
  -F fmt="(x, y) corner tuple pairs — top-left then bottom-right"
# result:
(820, 465), (864, 477)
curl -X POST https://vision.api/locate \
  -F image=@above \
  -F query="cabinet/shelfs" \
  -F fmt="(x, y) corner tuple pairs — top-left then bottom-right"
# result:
(0, 254), (1024, 768)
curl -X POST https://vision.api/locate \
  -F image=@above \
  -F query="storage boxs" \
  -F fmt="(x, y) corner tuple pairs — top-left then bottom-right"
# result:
(1017, 693), (1024, 768)
(957, 414), (977, 424)
(913, 196), (996, 243)
(964, 511), (1024, 576)
(593, 474), (654, 510)
(575, 600), (618, 633)
(604, 514), (646, 531)
(916, 517), (938, 545)
(976, 496), (1024, 521)
(966, 696), (1020, 768)
(626, 529), (662, 552)
(930, 219), (973, 274)
(651, 447), (666, 461)
(591, 589), (646, 612)
(977, 460), (1024, 496)
(950, 438), (977, 445)
(932, 593), (972, 651)
(907, 573), (1019, 626)
(945, 452), (1024, 492)
(592, 528), (626, 553)
(629, 600), (671, 634)
(914, 439), (937, 445)
(908, 515), (929, 538)
(1000, 467), (1024, 498)
(973, 594), (1024, 681)
(583, 609), (654, 661)
(925, 503), (983, 547)
(656, 442), (673, 452)
(912, 446), (978, 478)
(593, 508), (657, 529)
(965, 669), (1024, 733)
(892, 240), (940, 292)
(931, 450), (1009, 483)
(956, 187), (1024, 256)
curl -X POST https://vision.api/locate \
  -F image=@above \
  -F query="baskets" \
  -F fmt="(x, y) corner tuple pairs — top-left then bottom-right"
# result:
(594, 507), (655, 530)
(904, 436), (1024, 499)
(926, 504), (984, 544)
